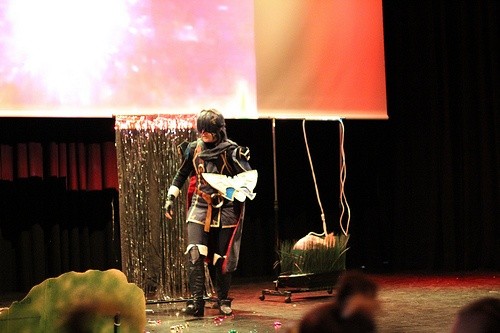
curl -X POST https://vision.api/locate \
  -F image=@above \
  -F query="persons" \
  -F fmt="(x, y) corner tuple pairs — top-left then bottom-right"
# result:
(451, 297), (500, 333)
(163, 109), (252, 318)
(298, 271), (378, 333)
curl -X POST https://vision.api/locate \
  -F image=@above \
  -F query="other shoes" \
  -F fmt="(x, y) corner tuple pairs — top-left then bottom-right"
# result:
(193, 299), (204, 317)
(218, 299), (233, 315)
